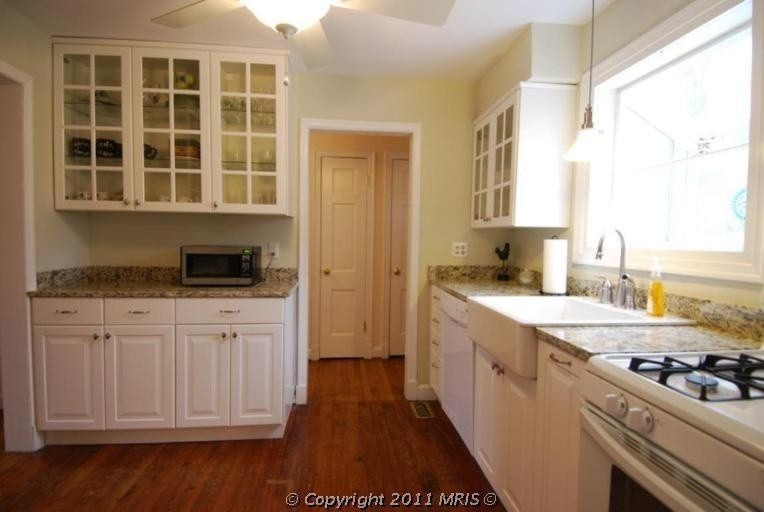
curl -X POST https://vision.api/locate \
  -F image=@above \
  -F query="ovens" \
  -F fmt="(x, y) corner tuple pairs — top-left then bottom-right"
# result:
(575, 369), (764, 512)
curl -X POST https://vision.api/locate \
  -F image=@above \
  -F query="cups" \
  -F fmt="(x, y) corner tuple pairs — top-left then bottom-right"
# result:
(74, 190), (90, 200)
(178, 195), (193, 203)
(98, 191), (110, 200)
(159, 195), (171, 202)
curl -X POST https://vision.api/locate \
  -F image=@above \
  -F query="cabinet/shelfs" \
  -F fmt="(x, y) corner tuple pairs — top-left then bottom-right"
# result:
(175, 285), (298, 429)
(529, 339), (611, 512)
(54, 42), (212, 211)
(427, 285), (439, 398)
(29, 296), (174, 431)
(212, 54), (289, 214)
(473, 345), (531, 511)
(469, 83), (573, 231)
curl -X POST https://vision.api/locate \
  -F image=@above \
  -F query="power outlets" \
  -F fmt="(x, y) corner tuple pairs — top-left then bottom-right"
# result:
(268, 241), (279, 259)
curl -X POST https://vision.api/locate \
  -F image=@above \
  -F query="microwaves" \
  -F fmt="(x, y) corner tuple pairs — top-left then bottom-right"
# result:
(180, 244), (263, 287)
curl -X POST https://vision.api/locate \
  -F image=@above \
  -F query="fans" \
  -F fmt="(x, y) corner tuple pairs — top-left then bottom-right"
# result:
(150, 1), (455, 33)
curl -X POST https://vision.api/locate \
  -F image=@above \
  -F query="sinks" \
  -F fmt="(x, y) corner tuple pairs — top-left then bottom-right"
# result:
(466, 296), (694, 378)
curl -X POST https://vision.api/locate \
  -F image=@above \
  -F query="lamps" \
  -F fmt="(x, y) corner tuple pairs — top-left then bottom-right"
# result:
(561, 2), (602, 161)
(245, 0), (331, 40)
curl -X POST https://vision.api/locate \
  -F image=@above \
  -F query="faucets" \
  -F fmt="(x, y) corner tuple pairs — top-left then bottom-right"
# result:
(591, 227), (627, 304)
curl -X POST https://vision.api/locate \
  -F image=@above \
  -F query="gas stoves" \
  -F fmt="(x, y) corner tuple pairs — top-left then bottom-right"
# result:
(583, 345), (764, 464)
